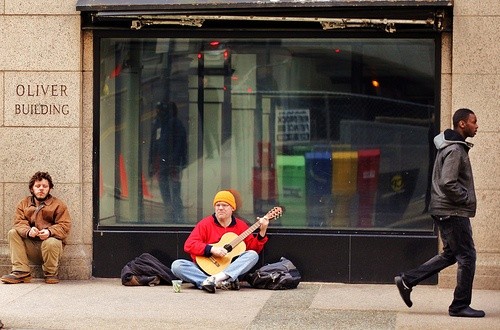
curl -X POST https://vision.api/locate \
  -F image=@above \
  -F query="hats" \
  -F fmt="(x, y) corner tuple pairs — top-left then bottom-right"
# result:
(213, 191), (237, 210)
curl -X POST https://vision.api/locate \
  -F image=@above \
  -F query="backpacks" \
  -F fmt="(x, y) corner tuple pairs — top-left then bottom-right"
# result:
(246, 256), (302, 290)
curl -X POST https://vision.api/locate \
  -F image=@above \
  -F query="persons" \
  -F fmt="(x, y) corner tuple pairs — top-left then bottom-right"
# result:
(150, 100), (185, 224)
(394, 108), (486, 318)
(1, 171), (72, 284)
(171, 191), (269, 293)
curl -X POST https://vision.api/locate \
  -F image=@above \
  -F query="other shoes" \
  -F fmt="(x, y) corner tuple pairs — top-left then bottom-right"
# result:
(1, 271), (32, 284)
(44, 273), (59, 284)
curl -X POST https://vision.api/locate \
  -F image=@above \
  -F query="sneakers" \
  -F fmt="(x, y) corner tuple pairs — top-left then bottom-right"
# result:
(220, 278), (240, 290)
(394, 275), (413, 308)
(199, 276), (216, 294)
(449, 300), (485, 317)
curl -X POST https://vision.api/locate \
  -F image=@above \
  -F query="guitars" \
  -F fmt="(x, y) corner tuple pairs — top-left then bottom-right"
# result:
(195, 206), (285, 276)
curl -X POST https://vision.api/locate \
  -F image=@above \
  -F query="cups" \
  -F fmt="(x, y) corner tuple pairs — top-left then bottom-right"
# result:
(172, 280), (183, 293)
